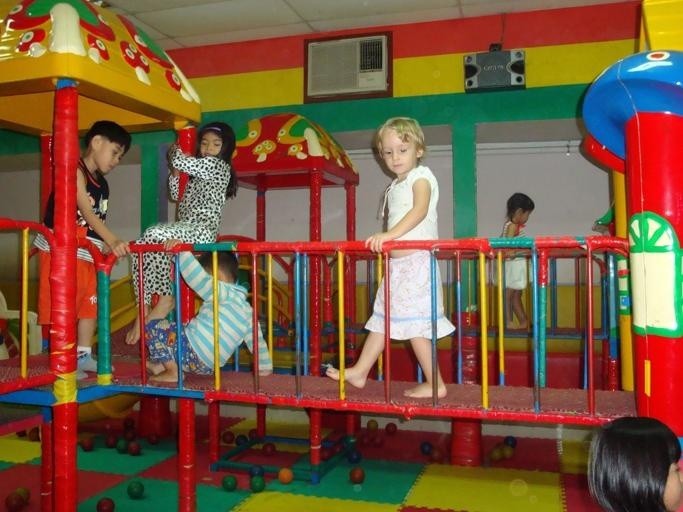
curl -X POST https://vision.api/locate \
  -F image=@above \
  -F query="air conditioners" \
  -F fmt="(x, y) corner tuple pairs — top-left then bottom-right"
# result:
(307, 35), (388, 97)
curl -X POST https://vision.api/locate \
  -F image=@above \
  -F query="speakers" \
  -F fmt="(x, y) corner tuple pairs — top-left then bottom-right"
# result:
(463, 49), (526, 93)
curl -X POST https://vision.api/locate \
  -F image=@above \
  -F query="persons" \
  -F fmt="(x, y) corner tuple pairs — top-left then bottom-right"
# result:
(587, 416), (683, 512)
(125, 122), (238, 346)
(143, 238), (273, 383)
(326, 117), (457, 399)
(33, 120), (131, 380)
(500, 193), (535, 329)
